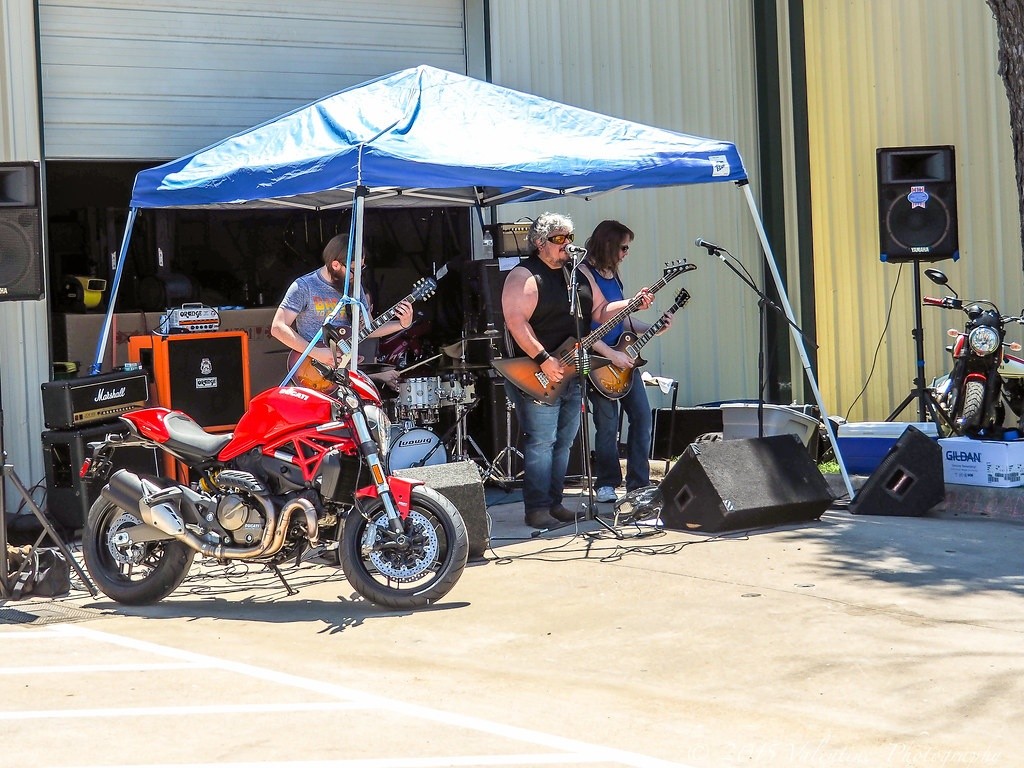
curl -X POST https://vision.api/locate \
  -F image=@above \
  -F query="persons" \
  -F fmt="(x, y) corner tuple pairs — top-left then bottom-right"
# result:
(357, 290), (401, 399)
(576, 218), (673, 503)
(500, 211), (656, 532)
(270, 234), (413, 370)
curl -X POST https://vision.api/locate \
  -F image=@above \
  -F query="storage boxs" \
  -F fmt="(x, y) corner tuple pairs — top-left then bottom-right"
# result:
(937, 435), (1024, 488)
(718, 402), (821, 450)
(836, 421), (938, 475)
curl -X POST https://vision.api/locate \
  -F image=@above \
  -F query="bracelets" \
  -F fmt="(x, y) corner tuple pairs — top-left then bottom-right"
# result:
(400, 323), (408, 329)
(533, 349), (550, 365)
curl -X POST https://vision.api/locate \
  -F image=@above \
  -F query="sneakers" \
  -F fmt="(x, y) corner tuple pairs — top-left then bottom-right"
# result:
(549, 503), (585, 520)
(595, 487), (618, 503)
(524, 509), (560, 530)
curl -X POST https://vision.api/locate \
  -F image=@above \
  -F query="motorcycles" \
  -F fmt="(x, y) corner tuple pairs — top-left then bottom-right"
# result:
(922, 267), (1024, 434)
(79, 358), (469, 609)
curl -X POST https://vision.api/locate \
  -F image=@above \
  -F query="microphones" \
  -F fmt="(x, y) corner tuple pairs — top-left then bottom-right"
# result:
(695, 238), (726, 251)
(564, 244), (586, 255)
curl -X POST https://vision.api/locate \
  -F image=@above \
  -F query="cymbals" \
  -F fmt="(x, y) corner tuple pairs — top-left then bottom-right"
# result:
(439, 363), (489, 369)
(455, 333), (503, 340)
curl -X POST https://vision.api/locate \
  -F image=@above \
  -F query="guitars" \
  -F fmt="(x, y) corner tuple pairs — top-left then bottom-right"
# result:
(286, 276), (437, 396)
(489, 259), (700, 408)
(587, 287), (692, 401)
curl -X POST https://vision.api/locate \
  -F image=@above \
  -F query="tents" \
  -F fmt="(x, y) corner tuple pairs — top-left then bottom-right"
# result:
(93, 66), (855, 505)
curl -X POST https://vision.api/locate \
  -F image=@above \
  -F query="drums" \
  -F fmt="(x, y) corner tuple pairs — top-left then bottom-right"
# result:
(438, 371), (477, 415)
(376, 422), (449, 475)
(389, 378), (443, 411)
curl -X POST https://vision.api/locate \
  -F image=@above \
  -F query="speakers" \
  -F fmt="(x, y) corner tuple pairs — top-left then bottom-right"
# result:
(159, 445), (204, 489)
(40, 421), (166, 530)
(129, 333), (251, 433)
(462, 254), (533, 371)
(655, 433), (837, 533)
(0, 161), (46, 301)
(391, 460), (494, 557)
(463, 370), (588, 485)
(875, 145), (959, 263)
(848, 425), (946, 517)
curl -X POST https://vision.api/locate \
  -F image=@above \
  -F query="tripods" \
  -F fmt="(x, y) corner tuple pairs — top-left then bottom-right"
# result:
(448, 401), (511, 493)
(885, 259), (963, 441)
(0, 376), (98, 601)
(479, 393), (529, 487)
(530, 254), (624, 538)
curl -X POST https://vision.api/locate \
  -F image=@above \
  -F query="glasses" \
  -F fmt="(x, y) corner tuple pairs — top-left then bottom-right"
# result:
(339, 260), (367, 275)
(542, 234), (575, 245)
(619, 244), (630, 253)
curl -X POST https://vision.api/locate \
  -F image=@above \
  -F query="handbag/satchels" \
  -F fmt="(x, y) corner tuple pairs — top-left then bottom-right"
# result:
(5, 542), (71, 600)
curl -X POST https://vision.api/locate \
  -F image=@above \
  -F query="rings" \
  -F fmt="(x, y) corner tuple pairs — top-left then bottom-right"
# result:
(647, 304), (653, 308)
(650, 302), (654, 305)
